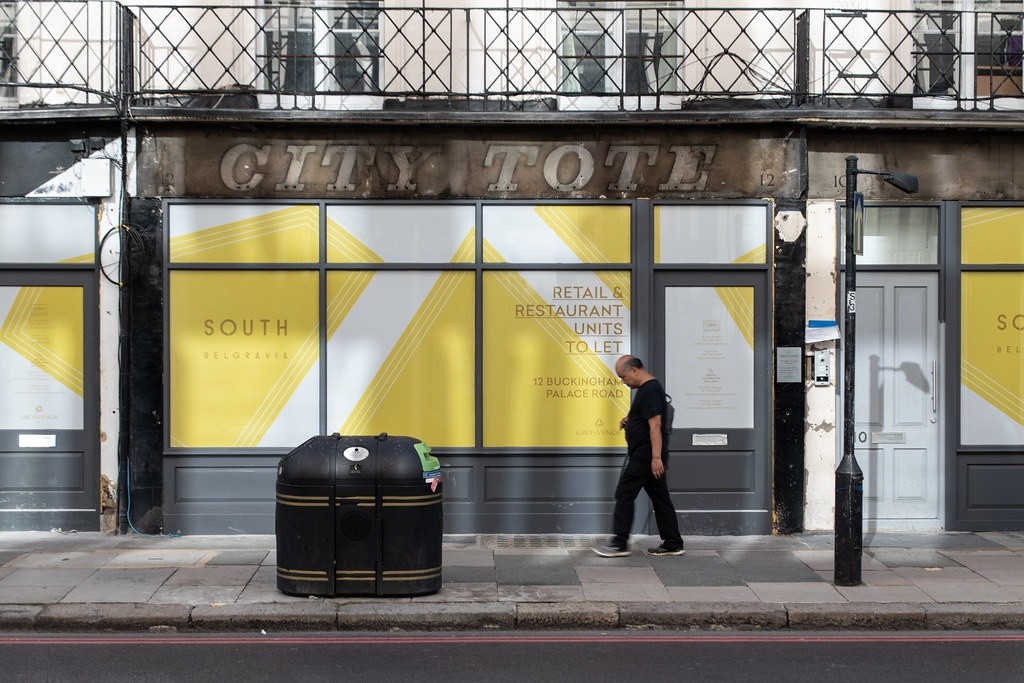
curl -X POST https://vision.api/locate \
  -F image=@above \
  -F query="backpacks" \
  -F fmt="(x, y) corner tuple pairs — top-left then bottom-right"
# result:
(665, 393), (675, 443)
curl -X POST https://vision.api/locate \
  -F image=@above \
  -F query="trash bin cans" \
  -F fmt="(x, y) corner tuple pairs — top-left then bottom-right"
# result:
(276, 434), (441, 593)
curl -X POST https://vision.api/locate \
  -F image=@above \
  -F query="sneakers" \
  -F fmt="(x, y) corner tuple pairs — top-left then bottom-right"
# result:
(591, 542), (631, 557)
(646, 544), (686, 556)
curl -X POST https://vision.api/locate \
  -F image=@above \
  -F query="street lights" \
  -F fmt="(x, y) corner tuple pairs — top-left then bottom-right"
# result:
(833, 154), (920, 588)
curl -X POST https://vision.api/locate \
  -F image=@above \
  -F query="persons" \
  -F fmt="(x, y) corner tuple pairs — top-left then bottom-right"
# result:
(590, 354), (685, 557)
